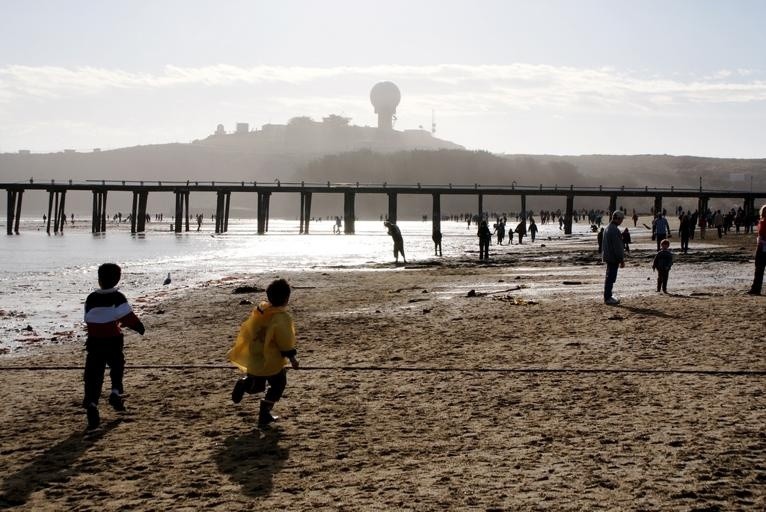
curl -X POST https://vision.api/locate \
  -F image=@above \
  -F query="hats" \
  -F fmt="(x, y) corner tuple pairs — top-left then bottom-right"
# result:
(614, 211), (624, 219)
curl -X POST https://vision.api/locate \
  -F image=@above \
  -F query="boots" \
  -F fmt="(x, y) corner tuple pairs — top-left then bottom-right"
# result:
(258, 400), (279, 425)
(232, 378), (245, 404)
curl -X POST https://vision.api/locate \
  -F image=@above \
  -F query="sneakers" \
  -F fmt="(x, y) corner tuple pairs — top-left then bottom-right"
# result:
(109, 392), (125, 411)
(606, 297), (621, 305)
(87, 402), (101, 426)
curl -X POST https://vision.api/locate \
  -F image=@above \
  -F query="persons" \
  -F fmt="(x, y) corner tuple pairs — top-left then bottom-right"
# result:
(650, 239), (675, 294)
(36, 210), (217, 232)
(441, 208), (566, 230)
(422, 214), (427, 221)
(432, 226), (442, 256)
(478, 219), (492, 261)
(79, 261), (145, 427)
(601, 208), (626, 305)
(652, 212), (671, 251)
(313, 214), (343, 234)
(745, 204), (766, 295)
(380, 214), (388, 220)
(678, 213), (692, 252)
(385, 222), (408, 263)
(494, 218), (538, 245)
(224, 276), (302, 427)
(571, 205), (761, 239)
